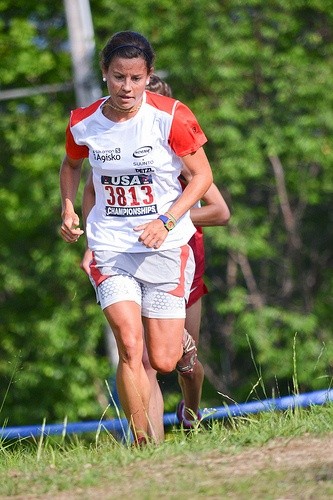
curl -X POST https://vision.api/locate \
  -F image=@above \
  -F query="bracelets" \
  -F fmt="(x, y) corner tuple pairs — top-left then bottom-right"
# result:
(166, 211), (178, 223)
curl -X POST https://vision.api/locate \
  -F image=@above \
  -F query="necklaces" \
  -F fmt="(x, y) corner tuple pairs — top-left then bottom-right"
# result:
(101, 99), (143, 113)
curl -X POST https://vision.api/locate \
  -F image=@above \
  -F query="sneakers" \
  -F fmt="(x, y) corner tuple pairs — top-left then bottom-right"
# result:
(176, 328), (198, 375)
(178, 400), (202, 434)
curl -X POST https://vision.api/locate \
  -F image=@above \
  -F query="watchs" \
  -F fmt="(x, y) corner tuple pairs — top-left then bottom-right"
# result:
(157, 214), (176, 232)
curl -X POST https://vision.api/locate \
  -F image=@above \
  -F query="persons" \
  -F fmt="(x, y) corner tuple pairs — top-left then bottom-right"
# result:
(58, 30), (214, 450)
(79, 73), (231, 446)
